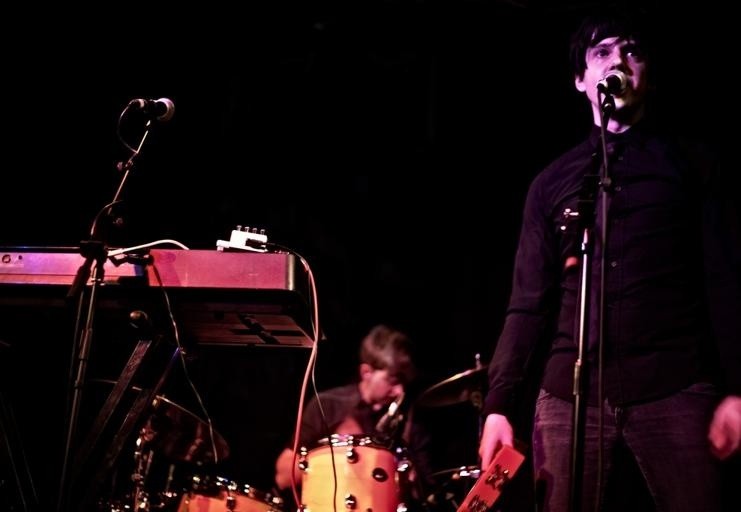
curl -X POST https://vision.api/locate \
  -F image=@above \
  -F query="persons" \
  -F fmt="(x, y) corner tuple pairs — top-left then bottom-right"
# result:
(477, 0), (741, 512)
(274, 326), (439, 491)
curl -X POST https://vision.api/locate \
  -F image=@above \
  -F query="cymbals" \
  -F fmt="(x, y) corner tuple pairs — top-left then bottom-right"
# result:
(419, 362), (491, 406)
(99, 379), (229, 462)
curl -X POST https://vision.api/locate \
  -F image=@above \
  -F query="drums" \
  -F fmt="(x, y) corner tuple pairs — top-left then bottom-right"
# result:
(178, 474), (287, 512)
(428, 464), (485, 510)
(297, 433), (417, 512)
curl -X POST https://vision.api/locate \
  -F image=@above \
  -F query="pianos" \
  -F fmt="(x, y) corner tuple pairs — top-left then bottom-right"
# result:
(0, 248), (296, 292)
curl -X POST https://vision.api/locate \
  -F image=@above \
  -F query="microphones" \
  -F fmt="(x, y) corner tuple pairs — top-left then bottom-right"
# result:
(596, 69), (626, 94)
(129, 98), (175, 118)
